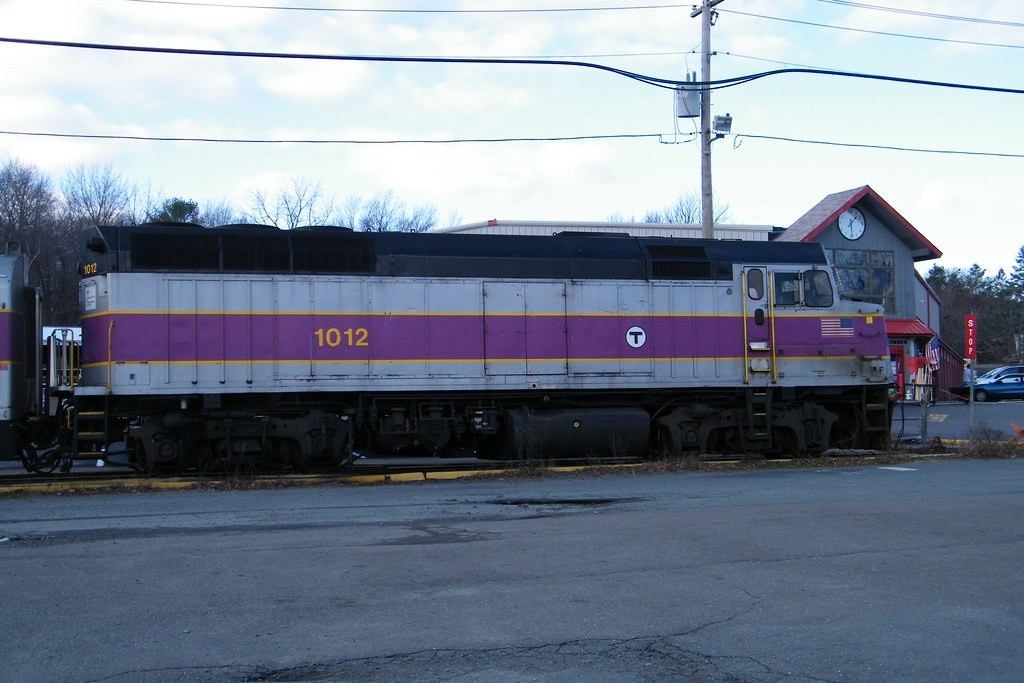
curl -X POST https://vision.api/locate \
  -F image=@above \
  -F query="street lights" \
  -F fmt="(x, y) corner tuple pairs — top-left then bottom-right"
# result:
(702, 113), (731, 239)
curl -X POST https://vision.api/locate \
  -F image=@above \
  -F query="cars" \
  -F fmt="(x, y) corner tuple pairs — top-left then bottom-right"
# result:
(962, 366), (1024, 402)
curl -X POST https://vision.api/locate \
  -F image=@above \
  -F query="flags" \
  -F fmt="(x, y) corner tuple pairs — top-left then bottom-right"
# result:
(925, 334), (941, 371)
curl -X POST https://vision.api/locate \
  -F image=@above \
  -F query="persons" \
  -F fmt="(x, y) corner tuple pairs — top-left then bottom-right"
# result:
(961, 364), (971, 387)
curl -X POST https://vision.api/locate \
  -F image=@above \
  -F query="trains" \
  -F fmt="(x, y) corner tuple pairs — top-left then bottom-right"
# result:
(1, 220), (900, 475)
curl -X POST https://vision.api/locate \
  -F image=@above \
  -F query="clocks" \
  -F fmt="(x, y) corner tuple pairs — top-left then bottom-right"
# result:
(836, 204), (868, 243)
